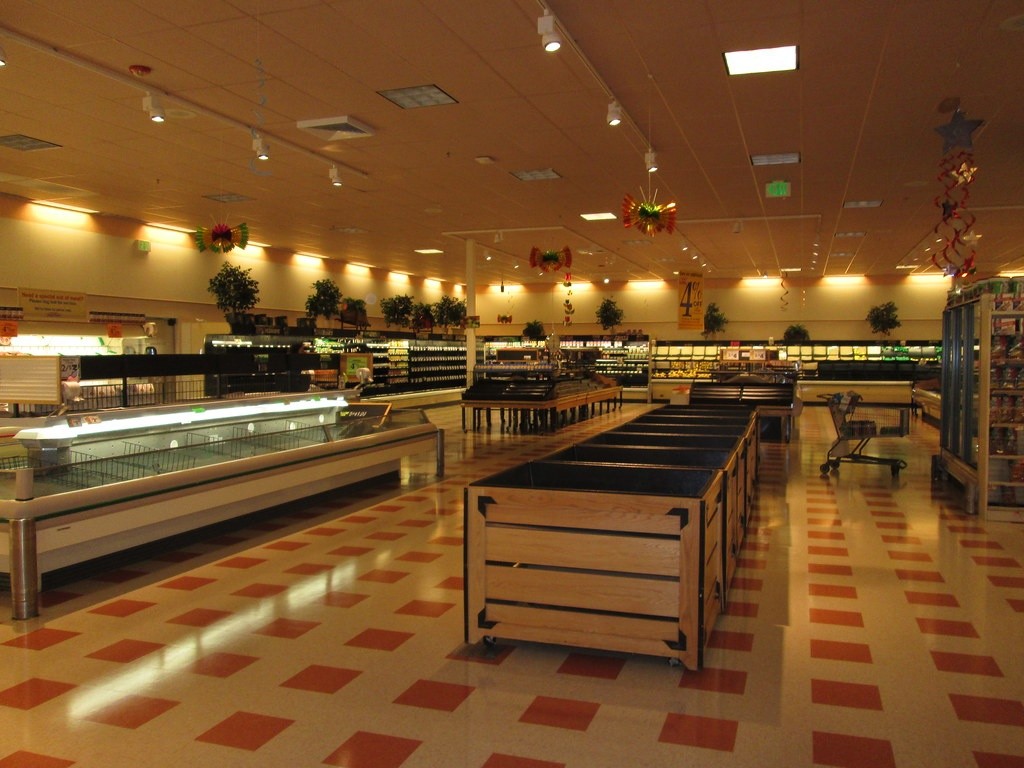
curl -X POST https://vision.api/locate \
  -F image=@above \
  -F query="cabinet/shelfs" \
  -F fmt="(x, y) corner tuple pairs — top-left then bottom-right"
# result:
(937, 294), (1024, 521)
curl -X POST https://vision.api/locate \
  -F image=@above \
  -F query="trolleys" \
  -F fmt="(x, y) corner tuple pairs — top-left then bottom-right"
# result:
(818, 392), (916, 478)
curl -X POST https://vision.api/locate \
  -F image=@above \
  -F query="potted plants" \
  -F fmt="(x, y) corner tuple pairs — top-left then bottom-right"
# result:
(521, 321), (544, 340)
(867, 302), (900, 339)
(209, 263), (466, 334)
(700, 303), (729, 340)
(595, 298), (622, 335)
(782, 324), (810, 343)
